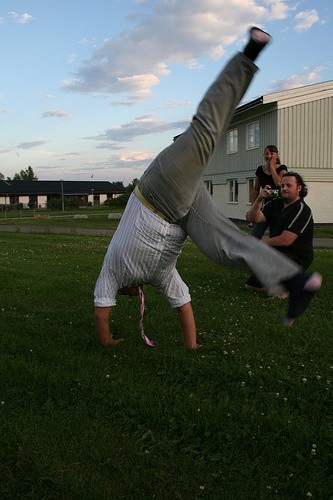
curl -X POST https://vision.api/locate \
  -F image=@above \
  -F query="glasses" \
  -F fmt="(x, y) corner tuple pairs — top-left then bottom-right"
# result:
(263, 152), (277, 156)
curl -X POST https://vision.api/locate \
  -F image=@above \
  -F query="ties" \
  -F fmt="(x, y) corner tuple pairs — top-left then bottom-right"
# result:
(138, 285), (159, 347)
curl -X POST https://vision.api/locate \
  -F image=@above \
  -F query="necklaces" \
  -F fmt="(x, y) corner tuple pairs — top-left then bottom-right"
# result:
(265, 163), (277, 173)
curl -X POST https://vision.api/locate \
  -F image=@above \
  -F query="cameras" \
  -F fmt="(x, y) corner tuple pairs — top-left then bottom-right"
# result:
(267, 189), (279, 197)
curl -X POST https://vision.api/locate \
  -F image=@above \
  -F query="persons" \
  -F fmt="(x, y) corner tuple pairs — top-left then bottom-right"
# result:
(93, 25), (324, 351)
(244, 171), (314, 300)
(250, 145), (288, 240)
